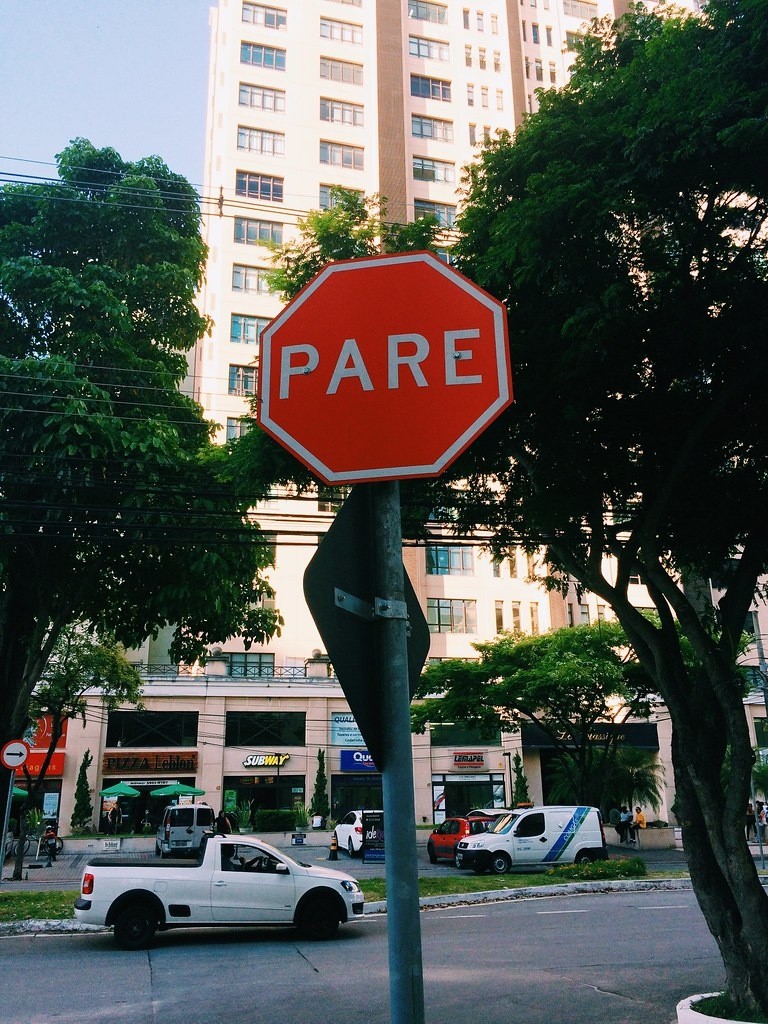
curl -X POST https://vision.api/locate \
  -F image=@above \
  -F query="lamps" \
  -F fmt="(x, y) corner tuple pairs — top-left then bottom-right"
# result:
(312, 649), (323, 660)
(211, 646), (223, 657)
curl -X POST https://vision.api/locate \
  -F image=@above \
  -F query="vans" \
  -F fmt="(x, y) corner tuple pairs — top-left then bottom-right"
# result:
(154, 804), (216, 858)
(456, 802), (608, 874)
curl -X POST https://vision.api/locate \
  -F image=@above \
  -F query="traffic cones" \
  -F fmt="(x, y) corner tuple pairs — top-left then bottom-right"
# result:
(327, 830), (339, 861)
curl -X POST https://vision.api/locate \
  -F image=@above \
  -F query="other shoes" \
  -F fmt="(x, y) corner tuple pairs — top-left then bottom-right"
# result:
(628, 840), (636, 844)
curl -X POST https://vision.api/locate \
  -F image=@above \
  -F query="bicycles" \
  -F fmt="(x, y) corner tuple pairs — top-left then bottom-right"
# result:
(14, 815), (63, 855)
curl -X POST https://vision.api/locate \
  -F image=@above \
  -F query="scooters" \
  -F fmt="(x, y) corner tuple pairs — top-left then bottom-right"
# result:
(41, 817), (60, 861)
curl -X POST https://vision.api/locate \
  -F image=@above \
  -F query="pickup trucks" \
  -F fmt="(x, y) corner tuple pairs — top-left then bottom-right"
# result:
(73, 830), (365, 951)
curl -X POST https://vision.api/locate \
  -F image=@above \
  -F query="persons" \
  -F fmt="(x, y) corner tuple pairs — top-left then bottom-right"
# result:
(215, 810), (232, 834)
(615, 806), (633, 843)
(108, 802), (122, 834)
(746, 800), (768, 844)
(629, 807), (646, 844)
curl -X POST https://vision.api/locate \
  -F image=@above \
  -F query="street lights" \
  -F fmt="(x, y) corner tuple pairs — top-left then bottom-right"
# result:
(503, 752), (513, 808)
(274, 753), (282, 810)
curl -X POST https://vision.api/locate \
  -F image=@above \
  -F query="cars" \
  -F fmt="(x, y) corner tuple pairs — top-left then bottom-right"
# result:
(427, 816), (498, 864)
(333, 809), (384, 858)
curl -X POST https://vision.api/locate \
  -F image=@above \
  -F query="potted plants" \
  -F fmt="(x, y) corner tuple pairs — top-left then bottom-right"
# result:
(26, 808), (47, 840)
(69, 747), (95, 836)
(231, 799), (255, 832)
(511, 747), (534, 808)
(293, 804), (317, 832)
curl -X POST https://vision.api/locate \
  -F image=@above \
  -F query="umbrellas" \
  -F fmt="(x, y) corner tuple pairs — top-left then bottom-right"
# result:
(99, 783), (141, 835)
(149, 783), (205, 805)
(12, 786), (28, 801)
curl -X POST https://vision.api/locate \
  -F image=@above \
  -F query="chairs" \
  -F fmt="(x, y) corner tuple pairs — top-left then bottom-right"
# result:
(221, 846), (239, 870)
